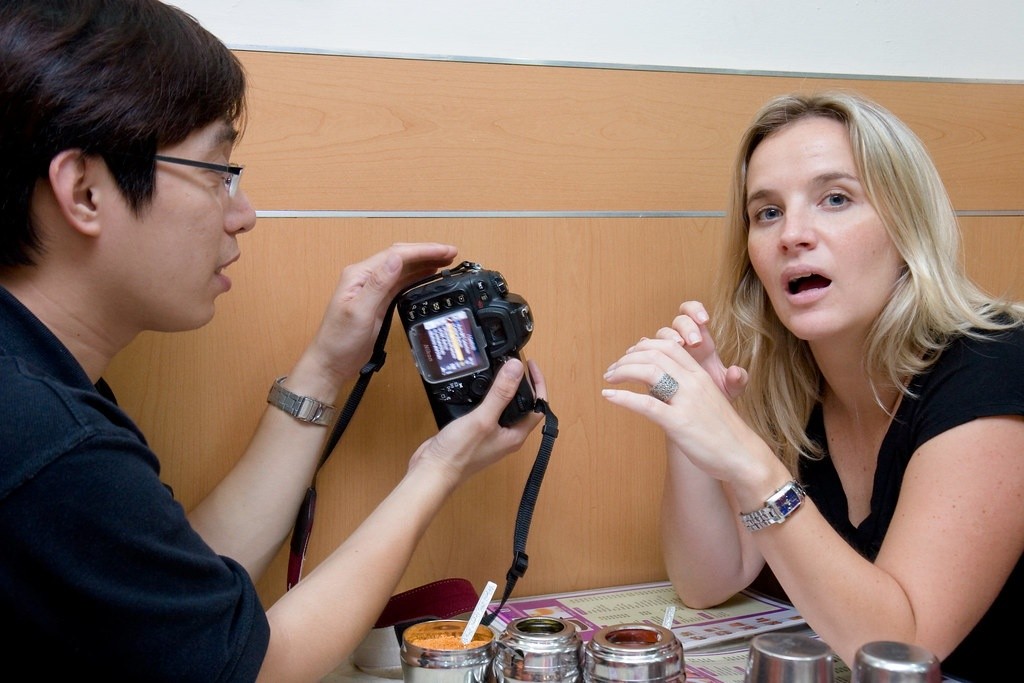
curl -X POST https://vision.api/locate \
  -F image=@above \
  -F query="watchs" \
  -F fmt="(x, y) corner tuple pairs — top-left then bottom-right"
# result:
(266, 375), (337, 428)
(739, 477), (806, 533)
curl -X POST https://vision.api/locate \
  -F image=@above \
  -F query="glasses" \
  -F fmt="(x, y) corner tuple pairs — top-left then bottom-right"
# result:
(156, 155), (245, 197)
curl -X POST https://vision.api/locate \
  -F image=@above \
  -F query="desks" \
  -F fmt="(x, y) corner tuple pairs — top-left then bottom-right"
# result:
(323, 578), (963, 683)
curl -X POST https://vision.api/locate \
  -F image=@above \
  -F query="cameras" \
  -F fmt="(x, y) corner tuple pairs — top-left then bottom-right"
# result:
(398, 267), (535, 433)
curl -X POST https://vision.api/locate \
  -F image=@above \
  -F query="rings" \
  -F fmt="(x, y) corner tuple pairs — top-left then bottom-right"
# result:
(649, 374), (678, 406)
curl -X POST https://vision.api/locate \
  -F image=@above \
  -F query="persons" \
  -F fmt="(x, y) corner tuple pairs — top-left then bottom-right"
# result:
(0, 0), (550, 683)
(601, 91), (1024, 683)
